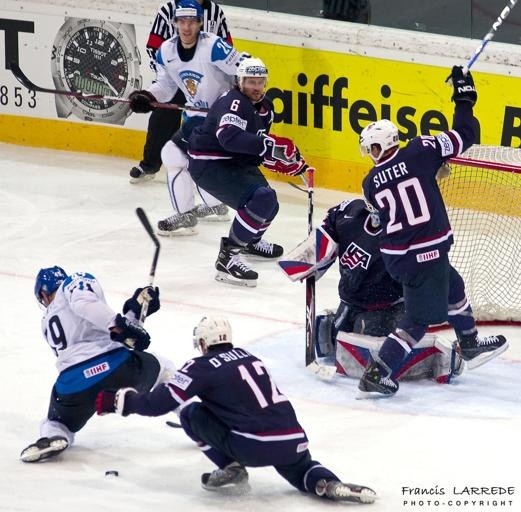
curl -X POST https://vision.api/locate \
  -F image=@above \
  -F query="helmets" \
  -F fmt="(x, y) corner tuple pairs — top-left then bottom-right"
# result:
(35, 266), (68, 310)
(358, 119), (400, 165)
(237, 57), (269, 103)
(173, 1), (204, 30)
(193, 316), (233, 355)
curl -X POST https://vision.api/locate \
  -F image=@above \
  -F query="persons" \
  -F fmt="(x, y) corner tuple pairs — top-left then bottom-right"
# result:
(127, 0), (250, 232)
(18, 266), (195, 462)
(127, 0), (233, 179)
(93, 312), (379, 506)
(186, 55), (308, 281)
(356, 66), (509, 395)
(275, 196), (465, 386)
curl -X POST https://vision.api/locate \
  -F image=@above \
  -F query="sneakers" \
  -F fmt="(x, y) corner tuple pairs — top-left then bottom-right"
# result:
(459, 331), (506, 361)
(21, 436), (68, 462)
(325, 481), (376, 504)
(195, 204), (228, 217)
(201, 465), (248, 487)
(358, 362), (398, 394)
(158, 211), (197, 231)
(130, 161), (160, 178)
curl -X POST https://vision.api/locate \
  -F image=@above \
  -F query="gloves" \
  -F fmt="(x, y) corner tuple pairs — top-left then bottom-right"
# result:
(259, 134), (300, 163)
(123, 286), (160, 320)
(128, 90), (158, 113)
(110, 314), (150, 351)
(451, 66), (477, 105)
(263, 155), (309, 177)
(95, 387), (138, 417)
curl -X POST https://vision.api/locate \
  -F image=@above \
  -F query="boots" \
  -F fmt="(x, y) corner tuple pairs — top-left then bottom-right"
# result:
(215, 237), (258, 279)
(240, 238), (283, 258)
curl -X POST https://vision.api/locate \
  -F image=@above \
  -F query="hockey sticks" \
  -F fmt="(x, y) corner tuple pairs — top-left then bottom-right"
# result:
(125, 207), (161, 349)
(8, 61), (209, 113)
